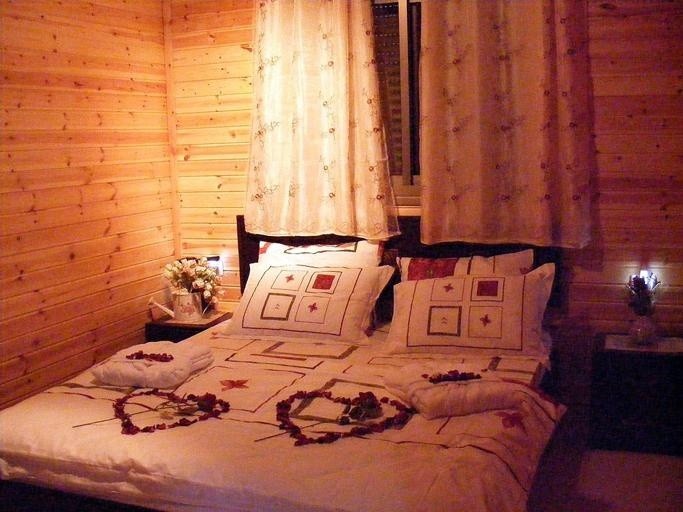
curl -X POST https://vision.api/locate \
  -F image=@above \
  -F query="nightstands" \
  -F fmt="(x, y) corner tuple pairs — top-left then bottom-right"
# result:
(588, 333), (682, 458)
(145, 310), (232, 343)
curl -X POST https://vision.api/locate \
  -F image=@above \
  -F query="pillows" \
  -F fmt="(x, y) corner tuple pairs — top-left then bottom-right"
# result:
(222, 237), (394, 363)
(378, 238), (557, 362)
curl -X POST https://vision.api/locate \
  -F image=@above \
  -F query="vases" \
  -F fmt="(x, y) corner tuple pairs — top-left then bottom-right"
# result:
(629, 316), (657, 345)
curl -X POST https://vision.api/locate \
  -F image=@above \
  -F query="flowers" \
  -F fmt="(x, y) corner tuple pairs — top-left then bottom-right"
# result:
(624, 269), (661, 315)
(161, 256), (224, 306)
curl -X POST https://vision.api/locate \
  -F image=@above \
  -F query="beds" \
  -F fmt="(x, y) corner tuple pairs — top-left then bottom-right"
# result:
(0, 214), (566, 512)
